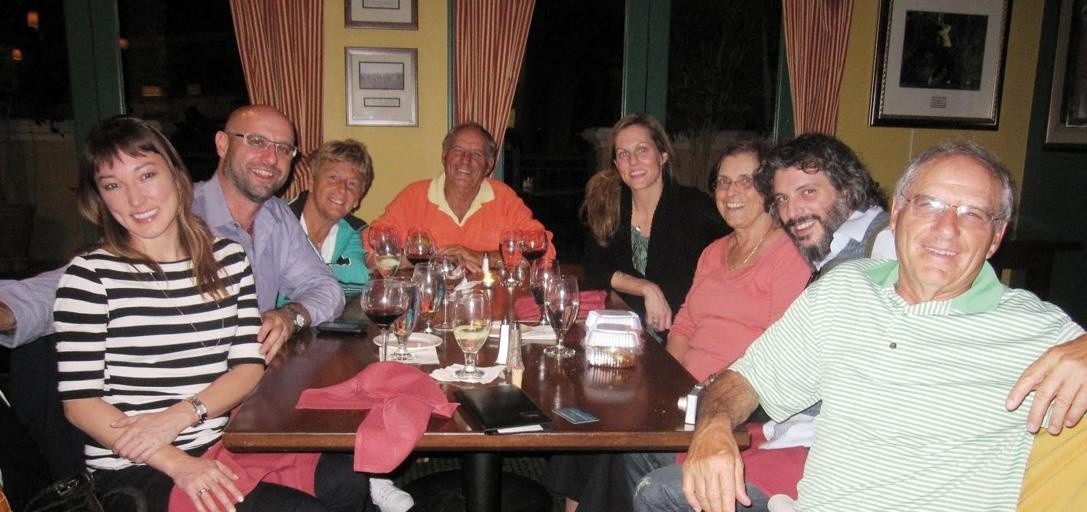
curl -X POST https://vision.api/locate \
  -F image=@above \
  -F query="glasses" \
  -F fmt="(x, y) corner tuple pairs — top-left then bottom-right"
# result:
(225, 133), (298, 158)
(900, 191), (1005, 226)
(712, 179), (755, 189)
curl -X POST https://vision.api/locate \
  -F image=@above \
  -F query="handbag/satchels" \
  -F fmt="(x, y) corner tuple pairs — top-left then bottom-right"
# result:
(22, 472), (104, 512)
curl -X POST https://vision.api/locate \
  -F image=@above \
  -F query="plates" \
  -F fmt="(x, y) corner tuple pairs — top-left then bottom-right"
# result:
(372, 332), (443, 349)
(488, 321), (530, 337)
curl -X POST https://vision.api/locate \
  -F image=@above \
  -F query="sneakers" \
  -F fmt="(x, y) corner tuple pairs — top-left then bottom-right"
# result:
(368, 478), (414, 511)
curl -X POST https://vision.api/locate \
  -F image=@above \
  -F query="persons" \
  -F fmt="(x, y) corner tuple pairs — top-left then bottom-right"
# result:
(361, 122), (556, 273)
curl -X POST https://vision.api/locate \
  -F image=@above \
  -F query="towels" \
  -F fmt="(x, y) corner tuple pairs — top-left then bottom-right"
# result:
(169, 407), (323, 512)
(514, 291), (608, 319)
(295, 362), (462, 473)
(672, 415), (806, 504)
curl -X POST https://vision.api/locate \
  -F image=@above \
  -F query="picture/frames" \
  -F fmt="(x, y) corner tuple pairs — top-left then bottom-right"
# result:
(344, 1), (418, 31)
(345, 46), (419, 128)
(866, 0), (1012, 130)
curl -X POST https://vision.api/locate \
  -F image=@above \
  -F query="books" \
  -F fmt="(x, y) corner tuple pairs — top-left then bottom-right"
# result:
(452, 384), (554, 437)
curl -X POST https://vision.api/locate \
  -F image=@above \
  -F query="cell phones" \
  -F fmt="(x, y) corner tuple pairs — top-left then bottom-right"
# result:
(317, 320), (369, 339)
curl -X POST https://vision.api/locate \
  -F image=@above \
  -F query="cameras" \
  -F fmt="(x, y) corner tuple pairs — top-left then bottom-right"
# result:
(678, 383), (705, 428)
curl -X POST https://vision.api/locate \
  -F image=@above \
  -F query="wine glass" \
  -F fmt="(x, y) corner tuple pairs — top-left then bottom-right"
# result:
(451, 288), (493, 377)
(518, 231), (548, 292)
(499, 229), (519, 288)
(368, 218), (403, 254)
(429, 248), (466, 332)
(360, 279), (411, 364)
(404, 224), (439, 264)
(412, 264), (446, 333)
(391, 282), (421, 361)
(531, 258), (562, 327)
(372, 235), (405, 302)
(544, 274), (580, 359)
